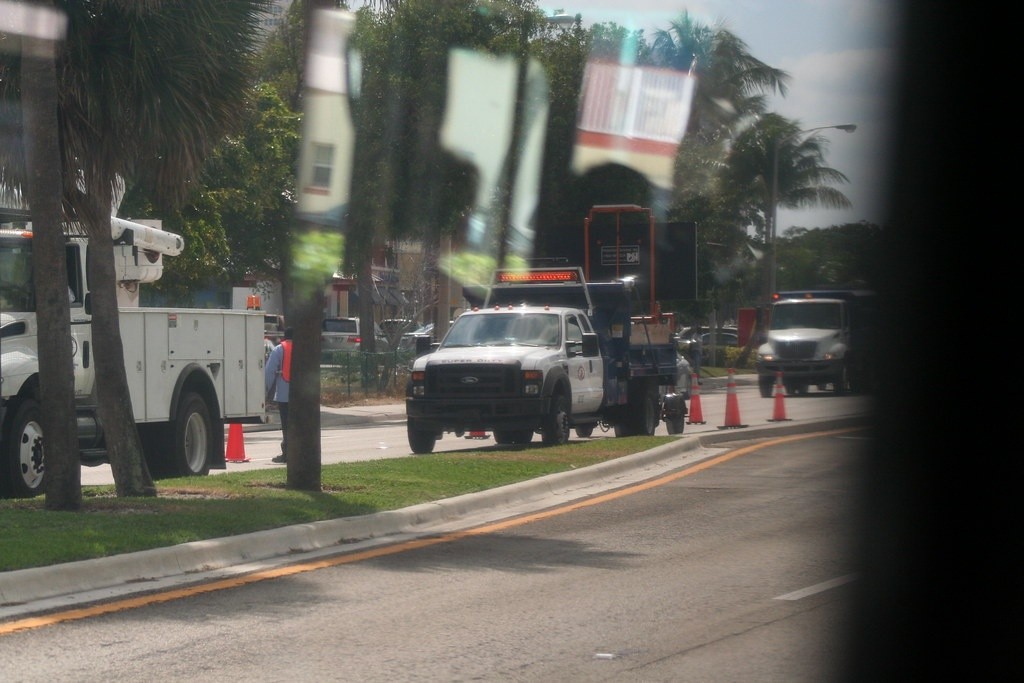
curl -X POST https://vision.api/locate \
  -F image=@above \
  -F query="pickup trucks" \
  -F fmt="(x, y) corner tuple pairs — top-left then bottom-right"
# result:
(265, 314), (286, 358)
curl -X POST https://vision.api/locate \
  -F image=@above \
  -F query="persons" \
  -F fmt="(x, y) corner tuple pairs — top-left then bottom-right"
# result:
(265, 327), (294, 463)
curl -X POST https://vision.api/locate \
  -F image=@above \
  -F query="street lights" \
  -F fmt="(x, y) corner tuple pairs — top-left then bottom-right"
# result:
(769, 123), (861, 302)
(692, 221), (727, 301)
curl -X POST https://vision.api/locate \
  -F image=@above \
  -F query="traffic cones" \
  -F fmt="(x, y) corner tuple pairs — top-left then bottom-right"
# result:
(715, 368), (750, 430)
(225, 423), (250, 464)
(684, 373), (708, 426)
(764, 372), (792, 421)
(463, 431), (490, 440)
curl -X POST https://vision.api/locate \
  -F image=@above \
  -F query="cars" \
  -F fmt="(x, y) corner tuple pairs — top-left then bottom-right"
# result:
(673, 323), (740, 348)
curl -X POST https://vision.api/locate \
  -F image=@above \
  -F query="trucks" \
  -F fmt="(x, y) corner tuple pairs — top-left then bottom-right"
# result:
(403, 266), (689, 454)
(756, 291), (864, 399)
(0, 162), (268, 500)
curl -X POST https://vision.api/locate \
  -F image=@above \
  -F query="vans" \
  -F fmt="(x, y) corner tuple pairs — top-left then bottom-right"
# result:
(378, 318), (425, 350)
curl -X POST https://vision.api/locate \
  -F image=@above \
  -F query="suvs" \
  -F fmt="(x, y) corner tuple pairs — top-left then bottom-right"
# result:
(318, 316), (391, 366)
(398, 320), (457, 364)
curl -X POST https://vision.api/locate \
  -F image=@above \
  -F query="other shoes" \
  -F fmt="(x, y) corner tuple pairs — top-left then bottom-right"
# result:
(272, 454), (288, 463)
